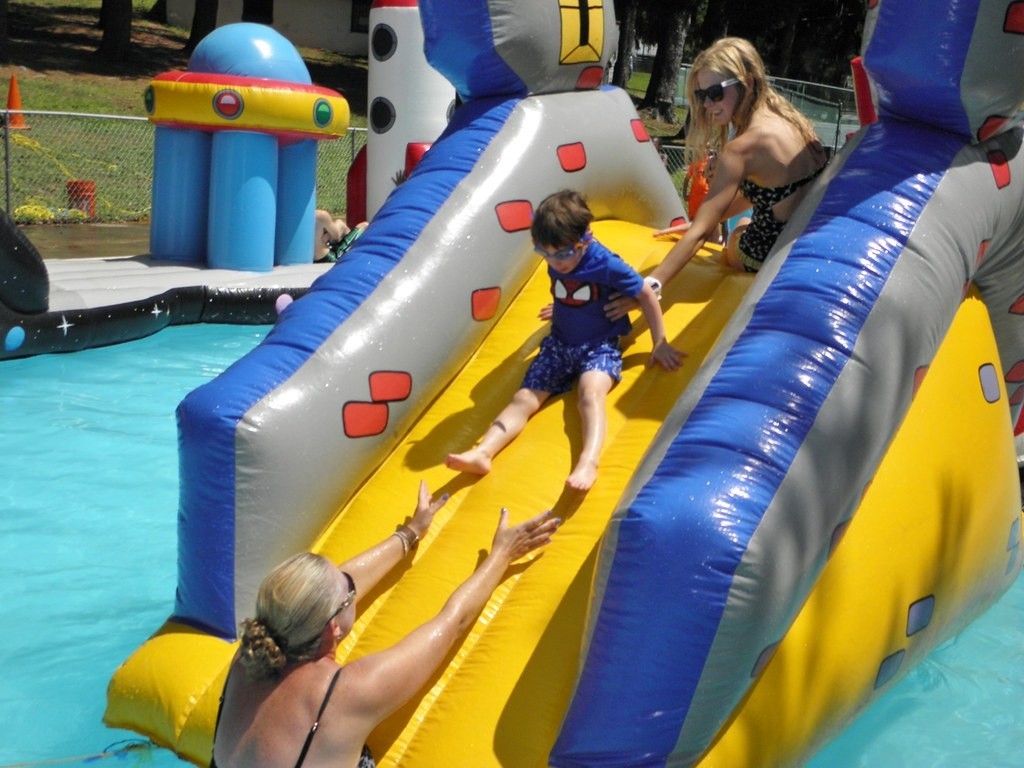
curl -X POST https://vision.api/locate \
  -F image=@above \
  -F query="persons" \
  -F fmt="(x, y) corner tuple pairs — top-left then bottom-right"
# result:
(445, 191), (689, 491)
(313, 209), (370, 264)
(605, 37), (831, 321)
(682, 140), (724, 246)
(209, 479), (561, 768)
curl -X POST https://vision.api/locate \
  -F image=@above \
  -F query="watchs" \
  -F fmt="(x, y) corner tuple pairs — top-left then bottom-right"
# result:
(644, 276), (662, 301)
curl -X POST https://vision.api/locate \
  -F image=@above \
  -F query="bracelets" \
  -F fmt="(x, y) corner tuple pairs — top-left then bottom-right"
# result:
(393, 533), (408, 556)
(399, 526), (419, 551)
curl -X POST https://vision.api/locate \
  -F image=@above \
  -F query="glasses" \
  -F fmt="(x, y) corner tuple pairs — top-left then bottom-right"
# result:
(534, 233), (592, 260)
(326, 572), (357, 625)
(693, 78), (740, 105)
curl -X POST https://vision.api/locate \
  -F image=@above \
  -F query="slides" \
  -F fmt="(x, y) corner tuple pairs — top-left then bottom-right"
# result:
(98, 86), (1024, 768)
